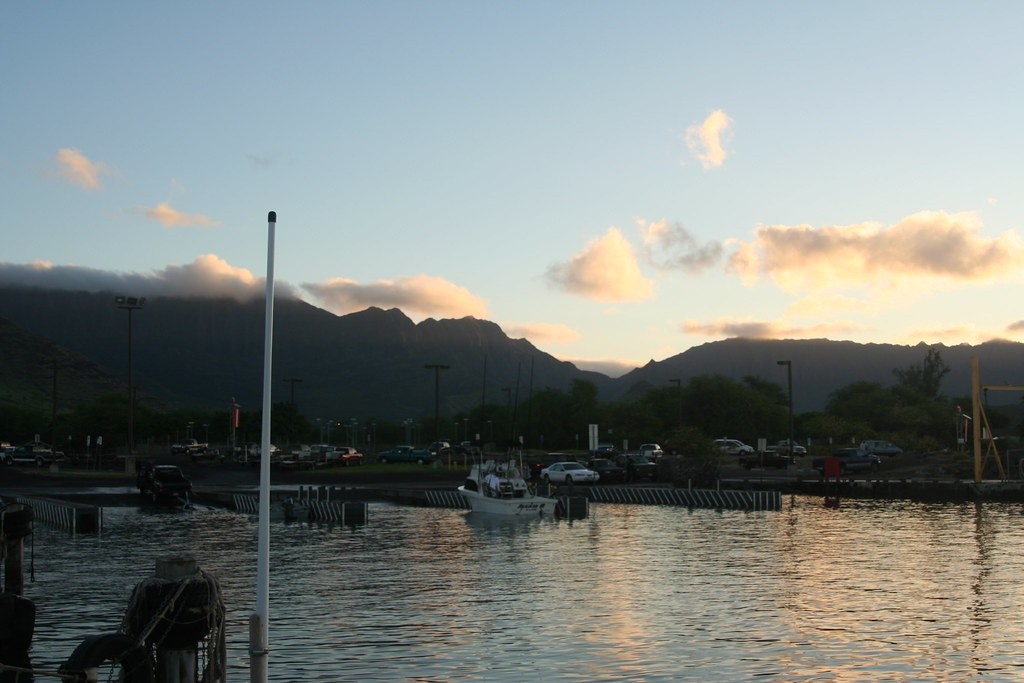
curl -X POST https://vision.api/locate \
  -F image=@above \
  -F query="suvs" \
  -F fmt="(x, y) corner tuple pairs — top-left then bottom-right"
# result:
(777, 440), (808, 457)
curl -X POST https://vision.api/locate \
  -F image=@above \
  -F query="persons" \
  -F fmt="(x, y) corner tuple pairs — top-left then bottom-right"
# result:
(860, 440), (868, 451)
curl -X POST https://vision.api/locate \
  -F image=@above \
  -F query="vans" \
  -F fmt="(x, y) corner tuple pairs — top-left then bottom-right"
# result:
(860, 440), (904, 457)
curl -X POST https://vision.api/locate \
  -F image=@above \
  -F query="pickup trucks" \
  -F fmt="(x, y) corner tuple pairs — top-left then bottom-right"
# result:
(813, 448), (881, 474)
(376, 445), (433, 465)
(170, 437), (208, 456)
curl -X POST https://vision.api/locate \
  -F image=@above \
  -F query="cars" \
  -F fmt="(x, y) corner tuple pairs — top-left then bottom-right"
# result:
(539, 462), (600, 485)
(280, 443), (366, 466)
(712, 439), (755, 456)
(4, 438), (66, 466)
(639, 443), (663, 460)
(586, 458), (626, 481)
(428, 441), (450, 456)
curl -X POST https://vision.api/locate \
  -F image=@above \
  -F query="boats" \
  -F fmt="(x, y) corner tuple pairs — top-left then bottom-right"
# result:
(457, 450), (559, 515)
